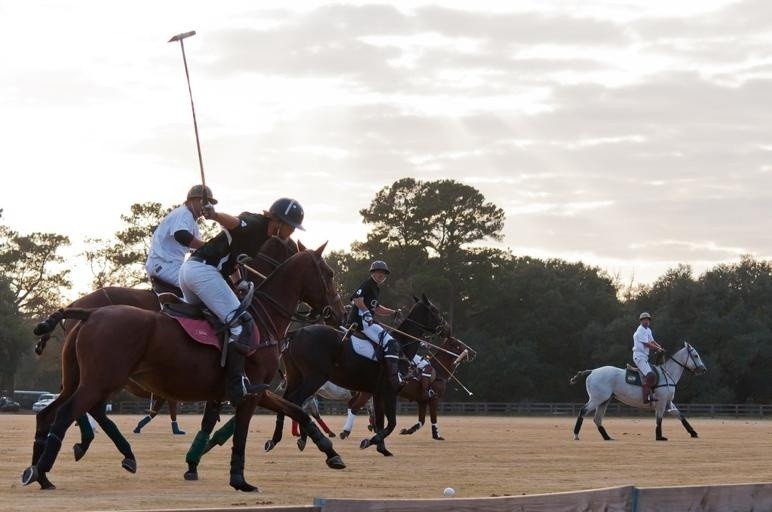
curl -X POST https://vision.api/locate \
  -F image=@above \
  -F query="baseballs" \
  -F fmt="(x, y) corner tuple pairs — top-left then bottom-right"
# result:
(443, 488), (455, 496)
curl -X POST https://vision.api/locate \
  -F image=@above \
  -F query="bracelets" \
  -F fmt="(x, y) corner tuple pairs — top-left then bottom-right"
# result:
(655, 346), (658, 351)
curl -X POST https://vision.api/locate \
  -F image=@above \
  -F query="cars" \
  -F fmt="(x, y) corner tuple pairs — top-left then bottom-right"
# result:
(0, 397), (19, 411)
(32, 399), (55, 411)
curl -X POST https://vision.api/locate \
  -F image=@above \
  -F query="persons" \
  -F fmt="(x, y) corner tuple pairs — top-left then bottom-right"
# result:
(146, 184), (217, 290)
(631, 312), (665, 404)
(179, 198), (306, 406)
(413, 354), (439, 401)
(347, 260), (413, 393)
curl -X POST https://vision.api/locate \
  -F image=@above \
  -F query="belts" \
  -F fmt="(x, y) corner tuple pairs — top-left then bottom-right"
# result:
(187, 257), (212, 265)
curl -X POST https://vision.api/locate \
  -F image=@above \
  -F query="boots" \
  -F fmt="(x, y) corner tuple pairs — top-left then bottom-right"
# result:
(227, 343), (270, 406)
(386, 356), (413, 391)
(421, 373), (438, 401)
(644, 385), (658, 404)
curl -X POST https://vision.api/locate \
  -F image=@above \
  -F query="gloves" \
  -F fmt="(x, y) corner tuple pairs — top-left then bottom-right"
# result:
(200, 203), (216, 220)
(655, 346), (663, 353)
(363, 311), (372, 326)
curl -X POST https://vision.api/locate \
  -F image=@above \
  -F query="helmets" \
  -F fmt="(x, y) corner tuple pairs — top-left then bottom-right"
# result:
(369, 261), (392, 272)
(639, 312), (651, 319)
(270, 197), (307, 231)
(187, 185), (218, 206)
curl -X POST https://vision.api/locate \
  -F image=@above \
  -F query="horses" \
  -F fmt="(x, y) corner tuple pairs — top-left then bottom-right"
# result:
(265, 294), (453, 456)
(34, 241), (301, 473)
(568, 340), (708, 441)
(122, 379), (187, 436)
(23, 239), (349, 492)
(339, 334), (477, 440)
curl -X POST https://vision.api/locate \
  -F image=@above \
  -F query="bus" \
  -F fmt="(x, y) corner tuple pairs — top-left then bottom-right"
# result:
(2, 390), (50, 410)
